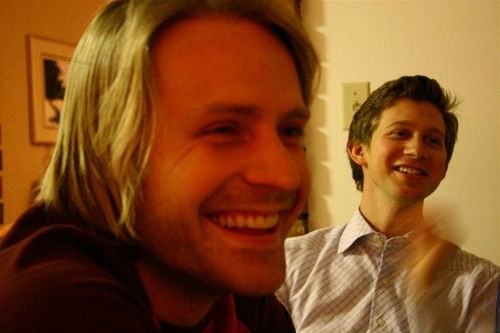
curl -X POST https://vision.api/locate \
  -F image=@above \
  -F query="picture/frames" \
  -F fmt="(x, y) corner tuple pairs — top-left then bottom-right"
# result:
(28, 34), (74, 146)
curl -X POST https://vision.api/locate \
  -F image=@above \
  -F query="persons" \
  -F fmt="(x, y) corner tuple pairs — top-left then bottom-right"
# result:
(273, 75), (500, 333)
(1, 0), (321, 333)
